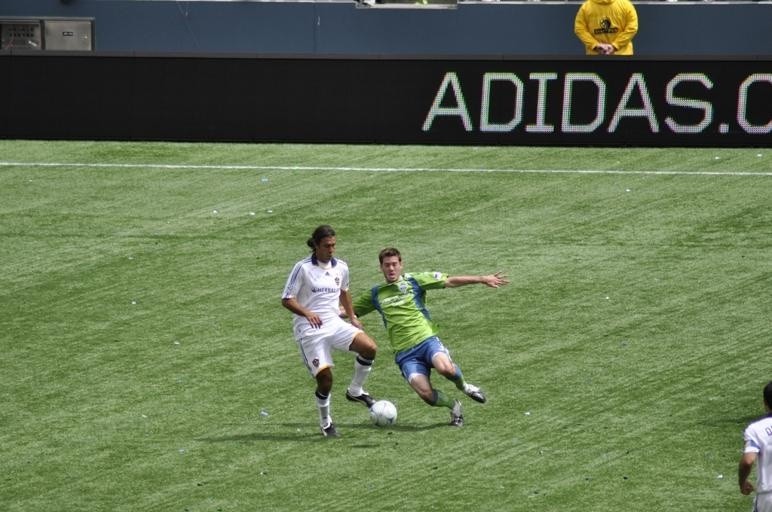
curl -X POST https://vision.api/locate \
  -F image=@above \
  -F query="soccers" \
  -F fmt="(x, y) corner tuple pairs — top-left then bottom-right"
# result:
(369, 400), (397, 426)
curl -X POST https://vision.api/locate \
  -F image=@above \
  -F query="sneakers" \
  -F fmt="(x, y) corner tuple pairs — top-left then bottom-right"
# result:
(320, 421), (341, 439)
(462, 383), (486, 403)
(450, 398), (464, 427)
(345, 389), (376, 409)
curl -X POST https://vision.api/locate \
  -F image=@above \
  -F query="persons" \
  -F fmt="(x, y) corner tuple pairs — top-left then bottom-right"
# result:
(281, 224), (378, 437)
(573, 0), (638, 56)
(737, 380), (772, 511)
(340, 247), (510, 426)
